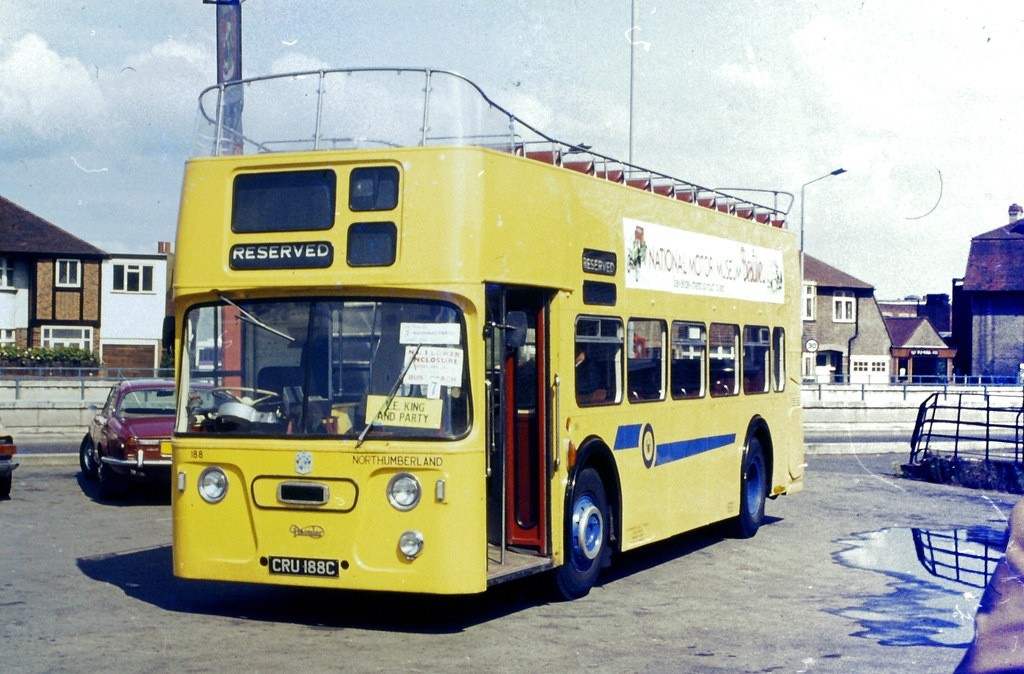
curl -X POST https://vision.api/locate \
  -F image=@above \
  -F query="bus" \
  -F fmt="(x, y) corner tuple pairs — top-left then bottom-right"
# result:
(159, 67), (811, 603)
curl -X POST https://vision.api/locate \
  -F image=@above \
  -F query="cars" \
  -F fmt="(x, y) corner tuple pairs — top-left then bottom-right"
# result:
(78, 378), (225, 501)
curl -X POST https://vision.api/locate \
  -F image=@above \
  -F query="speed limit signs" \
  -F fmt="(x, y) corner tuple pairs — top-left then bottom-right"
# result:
(805, 338), (820, 353)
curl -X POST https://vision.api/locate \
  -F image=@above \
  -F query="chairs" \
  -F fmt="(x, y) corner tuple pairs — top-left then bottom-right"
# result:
(258, 365), (315, 407)
(629, 369), (763, 402)
(482, 135), (788, 229)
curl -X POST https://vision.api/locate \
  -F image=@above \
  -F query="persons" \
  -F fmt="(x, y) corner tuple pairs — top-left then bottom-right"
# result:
(572, 342), (608, 403)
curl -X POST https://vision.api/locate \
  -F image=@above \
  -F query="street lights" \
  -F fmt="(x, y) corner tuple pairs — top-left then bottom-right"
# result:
(799, 168), (848, 279)
